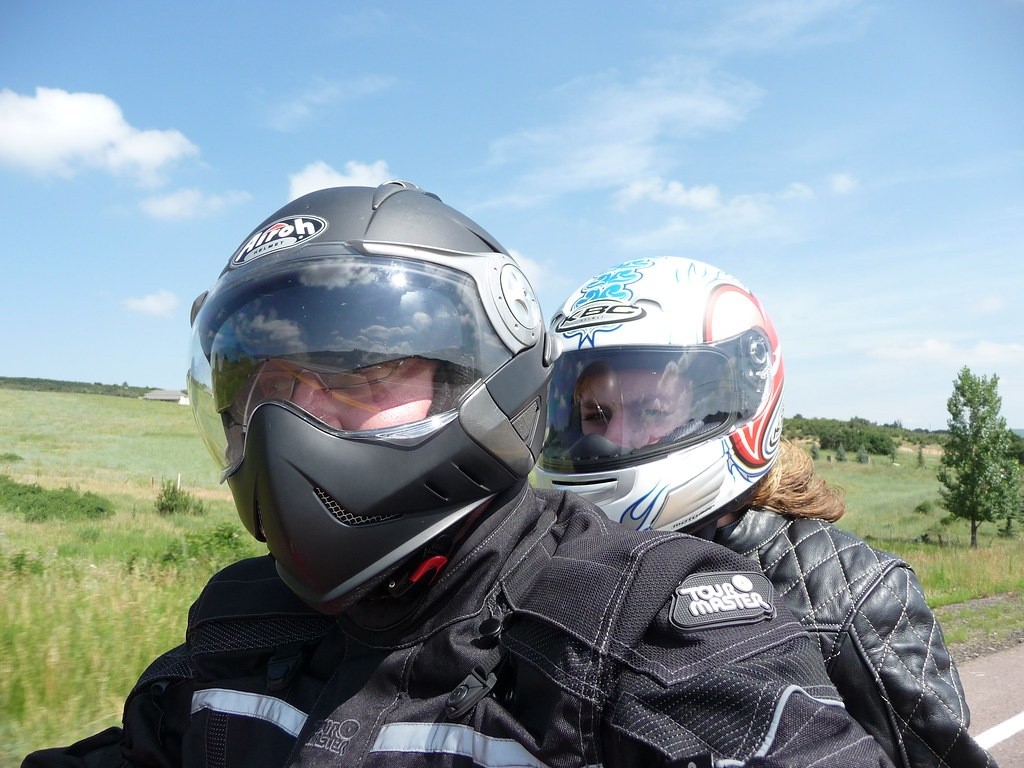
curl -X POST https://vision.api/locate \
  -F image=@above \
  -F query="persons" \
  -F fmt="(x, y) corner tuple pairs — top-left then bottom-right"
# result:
(12, 179), (896, 768)
(530, 250), (1007, 768)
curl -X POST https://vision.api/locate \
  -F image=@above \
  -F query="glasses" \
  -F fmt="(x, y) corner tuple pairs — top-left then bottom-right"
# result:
(235, 342), (405, 418)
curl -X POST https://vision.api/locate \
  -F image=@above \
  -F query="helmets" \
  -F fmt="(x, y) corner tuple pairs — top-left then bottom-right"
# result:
(535, 256), (784, 531)
(187, 180), (554, 616)
